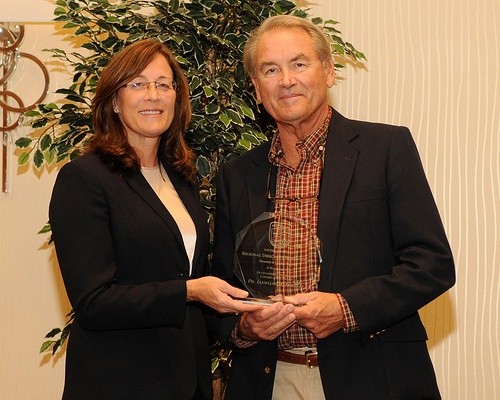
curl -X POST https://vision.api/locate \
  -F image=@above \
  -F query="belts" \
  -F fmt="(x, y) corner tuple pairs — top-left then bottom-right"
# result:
(277, 350), (319, 368)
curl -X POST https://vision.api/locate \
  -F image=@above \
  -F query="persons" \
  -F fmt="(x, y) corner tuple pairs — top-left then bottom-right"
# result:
(49, 36), (268, 400)
(209, 14), (455, 400)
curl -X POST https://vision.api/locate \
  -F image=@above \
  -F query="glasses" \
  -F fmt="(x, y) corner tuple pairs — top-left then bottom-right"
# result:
(124, 79), (178, 92)
